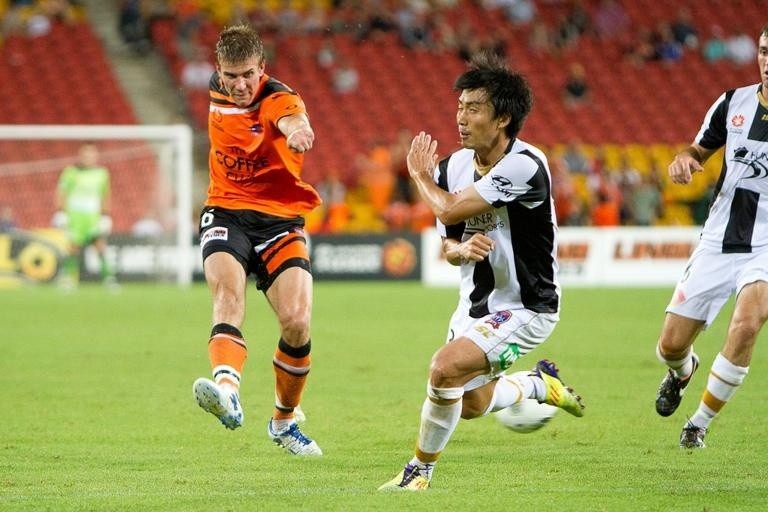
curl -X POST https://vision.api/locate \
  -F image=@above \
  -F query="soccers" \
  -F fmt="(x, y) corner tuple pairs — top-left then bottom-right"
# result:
(493, 371), (559, 434)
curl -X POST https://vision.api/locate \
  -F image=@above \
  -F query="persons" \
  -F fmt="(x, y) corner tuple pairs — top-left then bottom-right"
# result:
(193, 23), (324, 462)
(0, 2), (758, 283)
(378, 52), (586, 494)
(655, 27), (767, 450)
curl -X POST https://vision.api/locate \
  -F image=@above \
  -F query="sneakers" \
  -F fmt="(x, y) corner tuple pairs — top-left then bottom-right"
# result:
(527, 359), (585, 418)
(267, 417), (323, 458)
(654, 353), (701, 417)
(679, 416), (710, 449)
(376, 463), (431, 491)
(193, 377), (244, 430)
